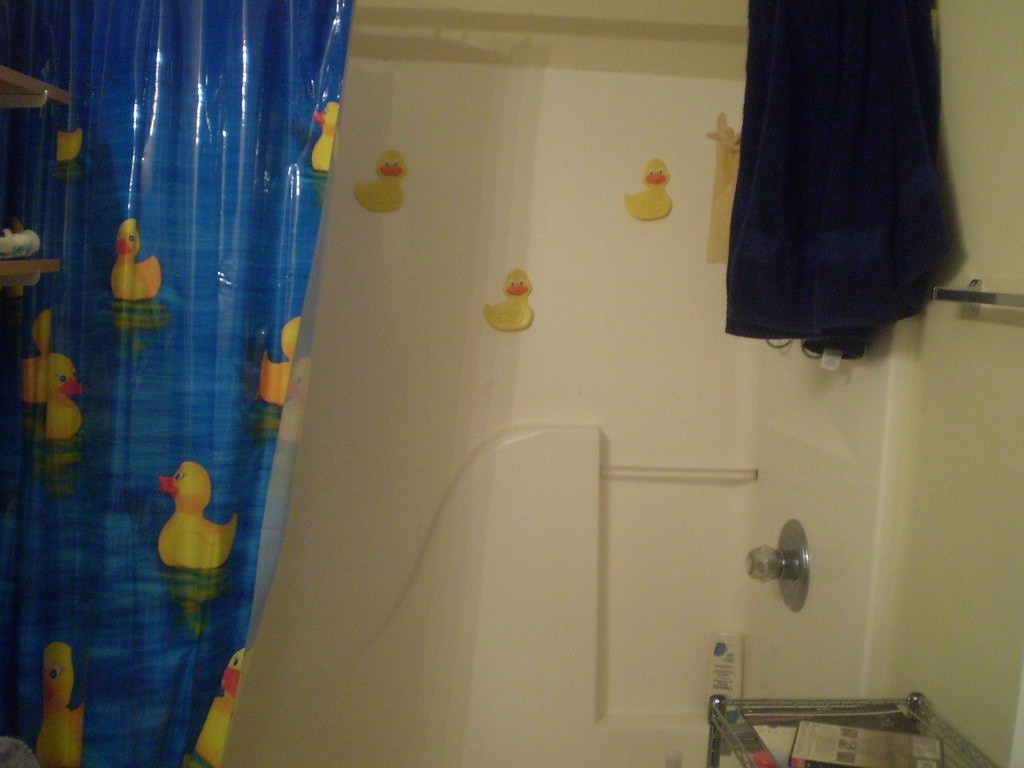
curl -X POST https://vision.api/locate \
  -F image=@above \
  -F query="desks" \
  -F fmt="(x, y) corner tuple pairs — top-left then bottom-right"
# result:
(706, 691), (1000, 768)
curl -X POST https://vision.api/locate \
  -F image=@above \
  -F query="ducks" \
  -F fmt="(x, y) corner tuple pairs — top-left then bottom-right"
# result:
(354, 148), (407, 214)
(625, 159), (674, 223)
(482, 268), (533, 333)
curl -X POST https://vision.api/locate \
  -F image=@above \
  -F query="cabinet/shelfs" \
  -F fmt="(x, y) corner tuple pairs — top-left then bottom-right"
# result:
(0, 63), (74, 298)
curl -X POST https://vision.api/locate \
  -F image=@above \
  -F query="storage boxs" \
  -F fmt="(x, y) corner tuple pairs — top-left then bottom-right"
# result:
(788, 719), (945, 768)
